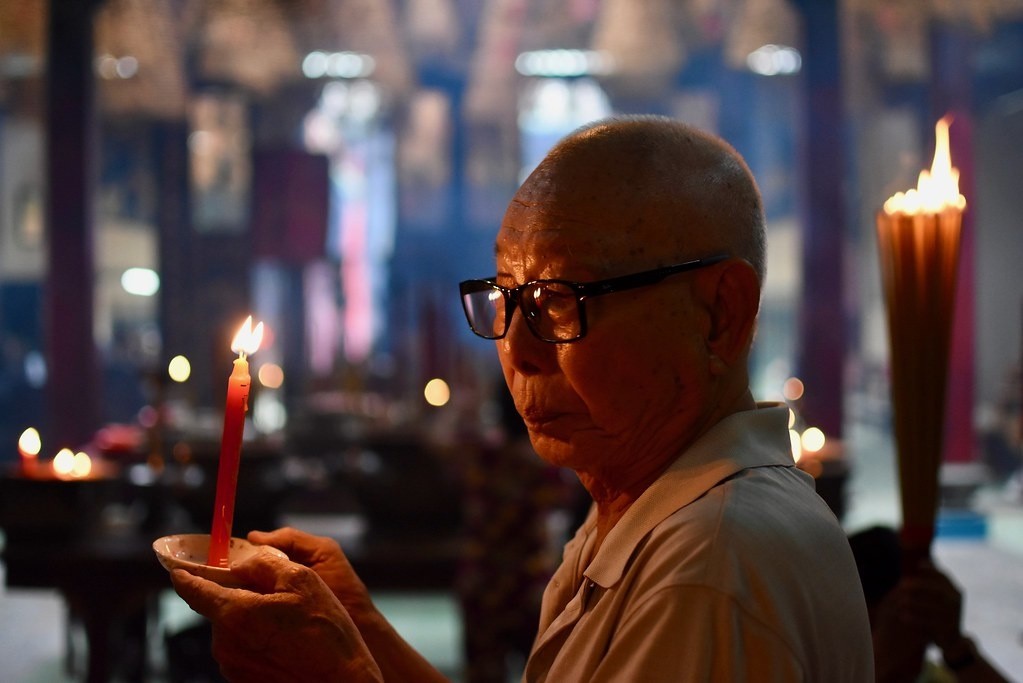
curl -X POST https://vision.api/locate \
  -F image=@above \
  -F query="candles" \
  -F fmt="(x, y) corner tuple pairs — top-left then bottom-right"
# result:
(208, 354), (250, 568)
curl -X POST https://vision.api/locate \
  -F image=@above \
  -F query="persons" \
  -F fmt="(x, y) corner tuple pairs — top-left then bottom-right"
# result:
(173, 114), (875, 683)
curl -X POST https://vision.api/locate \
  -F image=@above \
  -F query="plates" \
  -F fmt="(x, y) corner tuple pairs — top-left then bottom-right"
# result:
(153, 535), (289, 583)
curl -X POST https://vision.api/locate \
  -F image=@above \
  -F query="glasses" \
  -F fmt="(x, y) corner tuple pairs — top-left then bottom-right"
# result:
(458, 253), (734, 343)
